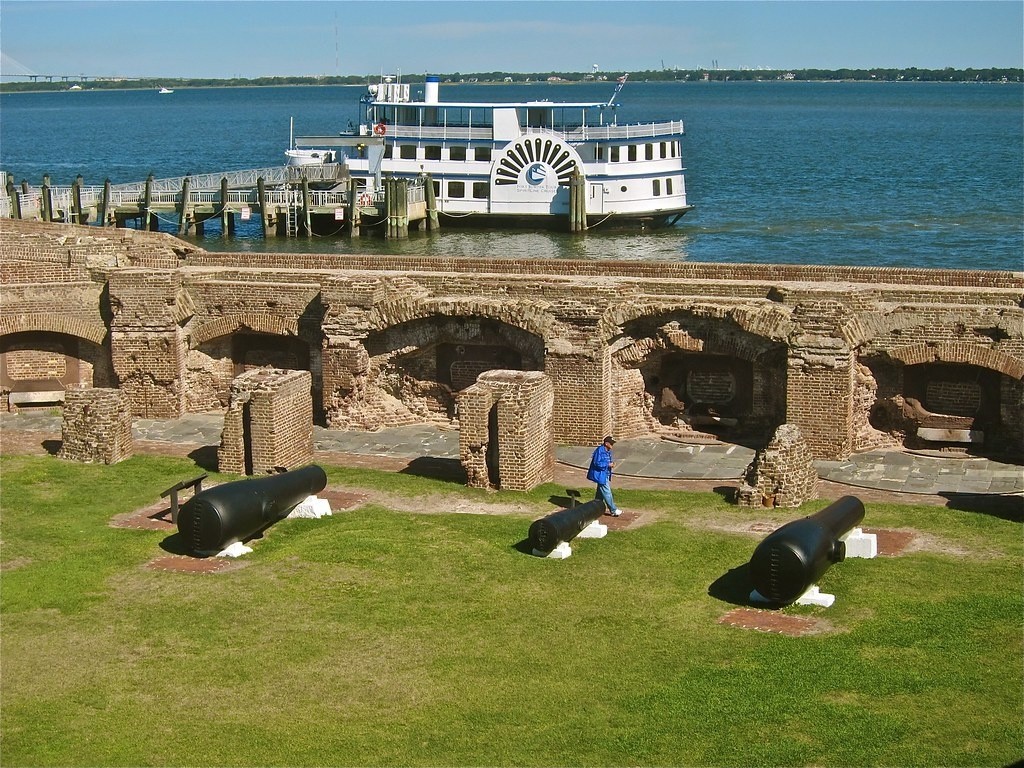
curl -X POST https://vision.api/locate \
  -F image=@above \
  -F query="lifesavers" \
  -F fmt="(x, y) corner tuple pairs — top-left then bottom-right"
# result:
(374, 124), (386, 135)
(360, 194), (371, 205)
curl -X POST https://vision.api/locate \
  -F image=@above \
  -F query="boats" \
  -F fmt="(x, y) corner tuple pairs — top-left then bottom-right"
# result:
(158, 85), (173, 93)
(282, 67), (696, 232)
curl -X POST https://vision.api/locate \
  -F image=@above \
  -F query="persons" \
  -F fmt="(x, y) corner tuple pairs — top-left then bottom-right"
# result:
(586, 435), (623, 518)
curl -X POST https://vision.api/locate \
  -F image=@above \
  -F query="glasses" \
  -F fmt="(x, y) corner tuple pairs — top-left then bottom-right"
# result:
(607, 442), (614, 445)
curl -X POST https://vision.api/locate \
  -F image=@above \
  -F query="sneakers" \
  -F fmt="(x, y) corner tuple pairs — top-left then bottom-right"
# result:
(611, 508), (623, 517)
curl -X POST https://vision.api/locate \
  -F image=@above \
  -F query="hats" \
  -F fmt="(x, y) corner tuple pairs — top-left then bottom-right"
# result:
(604, 436), (616, 444)
